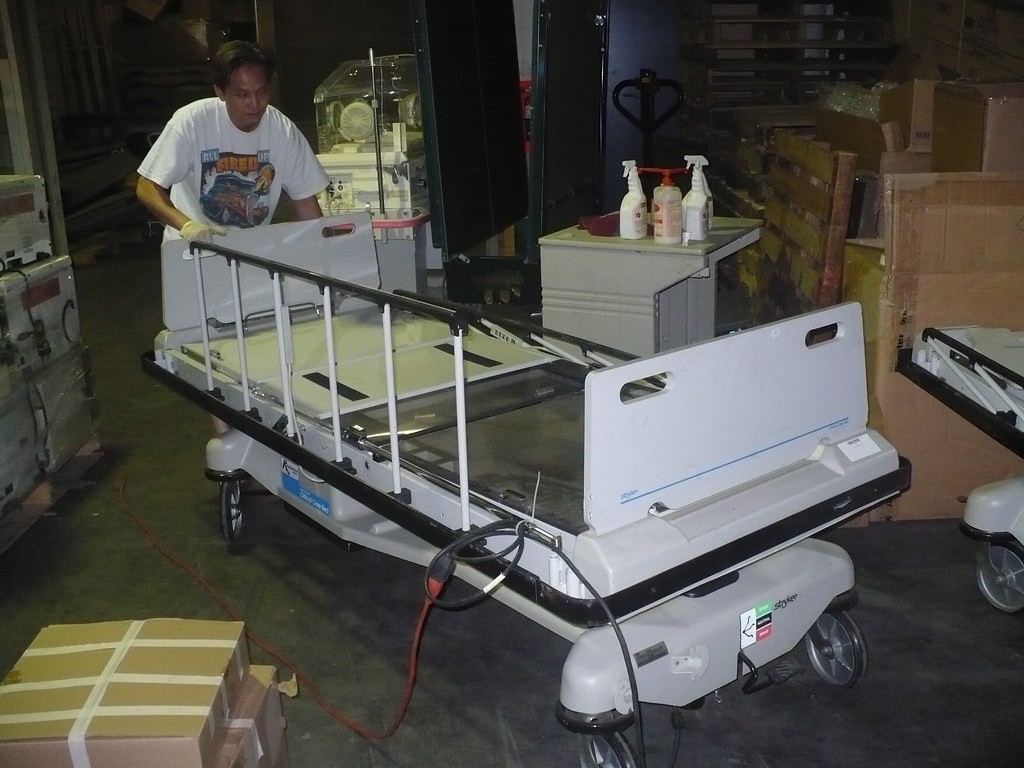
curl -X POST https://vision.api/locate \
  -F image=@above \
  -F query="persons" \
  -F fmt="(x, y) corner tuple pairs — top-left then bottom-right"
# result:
(135, 39), (325, 485)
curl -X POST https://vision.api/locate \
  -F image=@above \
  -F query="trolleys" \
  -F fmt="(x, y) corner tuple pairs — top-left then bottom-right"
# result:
(140, 207), (916, 768)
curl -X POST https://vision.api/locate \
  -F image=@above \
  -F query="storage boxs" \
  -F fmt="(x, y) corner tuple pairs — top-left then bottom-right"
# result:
(0, 618), (286, 768)
(812, 79), (1024, 523)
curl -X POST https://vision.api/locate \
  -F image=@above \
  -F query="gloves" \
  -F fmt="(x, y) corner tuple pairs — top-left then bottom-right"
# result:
(178, 220), (227, 245)
